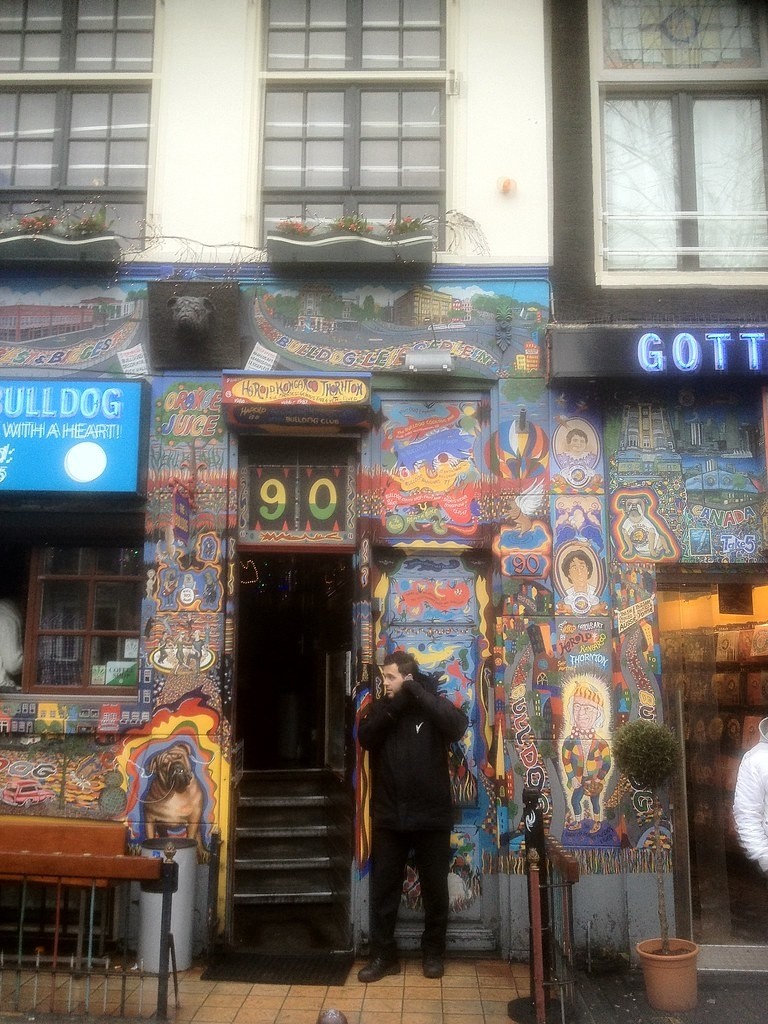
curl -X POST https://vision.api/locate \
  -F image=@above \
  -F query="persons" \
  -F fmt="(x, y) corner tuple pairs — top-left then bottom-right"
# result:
(42, 609), (99, 685)
(732, 716), (768, 875)
(355, 652), (470, 981)
(0, 597), (25, 688)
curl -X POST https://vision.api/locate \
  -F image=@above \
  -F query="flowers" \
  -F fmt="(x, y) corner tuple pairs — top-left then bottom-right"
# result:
(376, 212), (423, 234)
(68, 201), (115, 233)
(16, 214), (57, 234)
(274, 217), (323, 238)
(327, 208), (374, 236)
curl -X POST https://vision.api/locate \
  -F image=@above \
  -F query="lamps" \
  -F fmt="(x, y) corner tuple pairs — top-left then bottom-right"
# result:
(399, 350), (456, 376)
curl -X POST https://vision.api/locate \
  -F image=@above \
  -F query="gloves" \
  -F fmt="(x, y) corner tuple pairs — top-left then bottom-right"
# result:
(403, 679), (427, 702)
(387, 689), (412, 717)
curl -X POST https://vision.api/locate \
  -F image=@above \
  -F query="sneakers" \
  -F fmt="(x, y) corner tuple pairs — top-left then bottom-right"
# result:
(422, 953), (445, 979)
(357, 958), (401, 983)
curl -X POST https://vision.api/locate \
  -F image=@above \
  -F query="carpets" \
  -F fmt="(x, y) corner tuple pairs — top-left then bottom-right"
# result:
(200, 952), (358, 987)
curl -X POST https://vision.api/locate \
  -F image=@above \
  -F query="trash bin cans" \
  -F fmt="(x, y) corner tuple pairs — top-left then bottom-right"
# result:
(135, 837), (199, 974)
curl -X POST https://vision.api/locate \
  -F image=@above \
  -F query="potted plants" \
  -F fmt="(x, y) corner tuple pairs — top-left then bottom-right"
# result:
(610, 718), (701, 1012)
